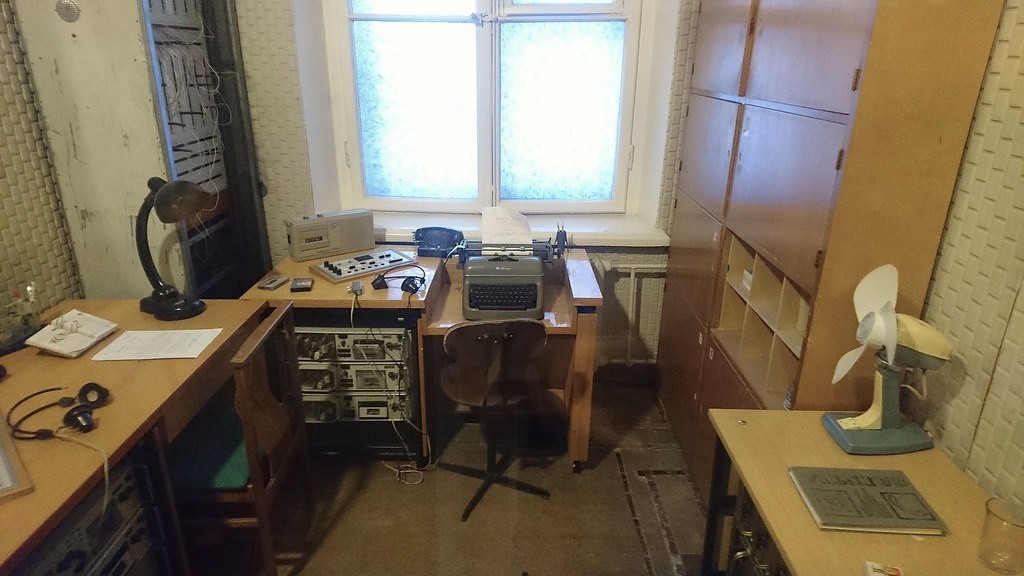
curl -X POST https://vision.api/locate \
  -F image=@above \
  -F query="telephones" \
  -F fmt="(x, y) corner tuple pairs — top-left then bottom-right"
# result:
(412, 227), (464, 258)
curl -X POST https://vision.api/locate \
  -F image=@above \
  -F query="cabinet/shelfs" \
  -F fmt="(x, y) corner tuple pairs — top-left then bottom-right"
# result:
(654, 271), (766, 511)
(678, 93), (968, 295)
(689, 0), (1005, 126)
(717, 235), (926, 411)
(187, 218), (241, 294)
(666, 189), (732, 329)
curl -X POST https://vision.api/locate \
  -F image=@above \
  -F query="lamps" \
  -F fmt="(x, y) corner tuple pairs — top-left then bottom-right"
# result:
(134, 177), (210, 321)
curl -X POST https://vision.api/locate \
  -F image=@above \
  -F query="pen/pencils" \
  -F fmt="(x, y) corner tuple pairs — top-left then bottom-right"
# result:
(26, 281), (36, 304)
(556, 221), (560, 232)
(562, 221), (564, 233)
(15, 289), (22, 303)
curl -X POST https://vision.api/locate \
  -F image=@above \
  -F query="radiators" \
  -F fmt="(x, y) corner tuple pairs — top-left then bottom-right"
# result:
(588, 254), (612, 372)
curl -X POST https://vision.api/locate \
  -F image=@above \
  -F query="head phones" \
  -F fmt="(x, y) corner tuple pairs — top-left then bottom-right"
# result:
(5, 382), (109, 439)
(371, 263), (426, 294)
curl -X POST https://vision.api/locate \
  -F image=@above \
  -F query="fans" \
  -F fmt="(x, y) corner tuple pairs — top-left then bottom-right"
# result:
(821, 263), (953, 455)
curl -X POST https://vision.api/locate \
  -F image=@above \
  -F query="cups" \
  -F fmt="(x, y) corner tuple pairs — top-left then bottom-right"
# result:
(978, 497), (1024, 575)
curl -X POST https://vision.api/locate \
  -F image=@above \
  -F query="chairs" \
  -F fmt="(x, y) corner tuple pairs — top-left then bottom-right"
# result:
(435, 318), (552, 522)
(170, 299), (310, 576)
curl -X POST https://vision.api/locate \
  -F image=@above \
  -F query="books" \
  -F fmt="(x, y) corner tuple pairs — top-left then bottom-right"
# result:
(25, 309), (118, 358)
(788, 467), (947, 536)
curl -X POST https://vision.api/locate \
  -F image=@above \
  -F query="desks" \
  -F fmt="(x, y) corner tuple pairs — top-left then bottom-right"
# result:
(701, 409), (1024, 576)
(0, 299), (271, 576)
(237, 244), (441, 460)
(422, 249), (604, 474)
